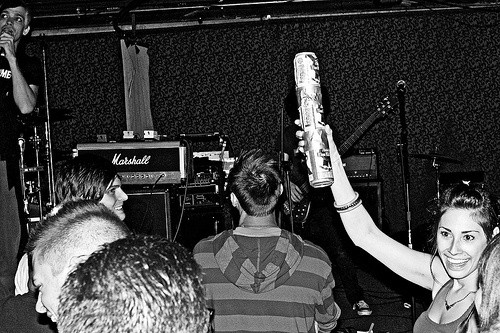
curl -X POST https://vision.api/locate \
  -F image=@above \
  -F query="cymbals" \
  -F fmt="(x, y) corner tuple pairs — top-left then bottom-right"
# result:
(28, 107), (73, 116)
(413, 153), (462, 164)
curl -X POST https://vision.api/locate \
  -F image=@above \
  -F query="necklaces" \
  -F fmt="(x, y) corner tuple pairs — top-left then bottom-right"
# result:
(444, 286), (471, 310)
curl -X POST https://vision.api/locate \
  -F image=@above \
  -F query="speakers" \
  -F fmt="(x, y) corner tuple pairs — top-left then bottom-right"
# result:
(121, 190), (171, 243)
(278, 180), (383, 290)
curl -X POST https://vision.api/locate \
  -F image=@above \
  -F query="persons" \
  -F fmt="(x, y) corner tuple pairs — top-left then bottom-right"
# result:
(26, 199), (134, 325)
(0, 0), (40, 303)
(193, 145), (340, 333)
(14, 155), (128, 298)
(293, 119), (500, 333)
(56, 233), (212, 333)
(276, 85), (372, 316)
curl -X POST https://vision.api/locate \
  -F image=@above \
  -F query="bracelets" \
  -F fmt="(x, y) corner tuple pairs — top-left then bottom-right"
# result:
(333, 191), (362, 213)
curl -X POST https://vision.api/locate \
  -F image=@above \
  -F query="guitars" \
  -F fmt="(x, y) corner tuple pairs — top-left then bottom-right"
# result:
(275, 93), (397, 223)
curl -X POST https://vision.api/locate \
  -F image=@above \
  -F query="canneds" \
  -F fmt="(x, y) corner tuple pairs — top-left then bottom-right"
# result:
(294, 52), (334, 188)
